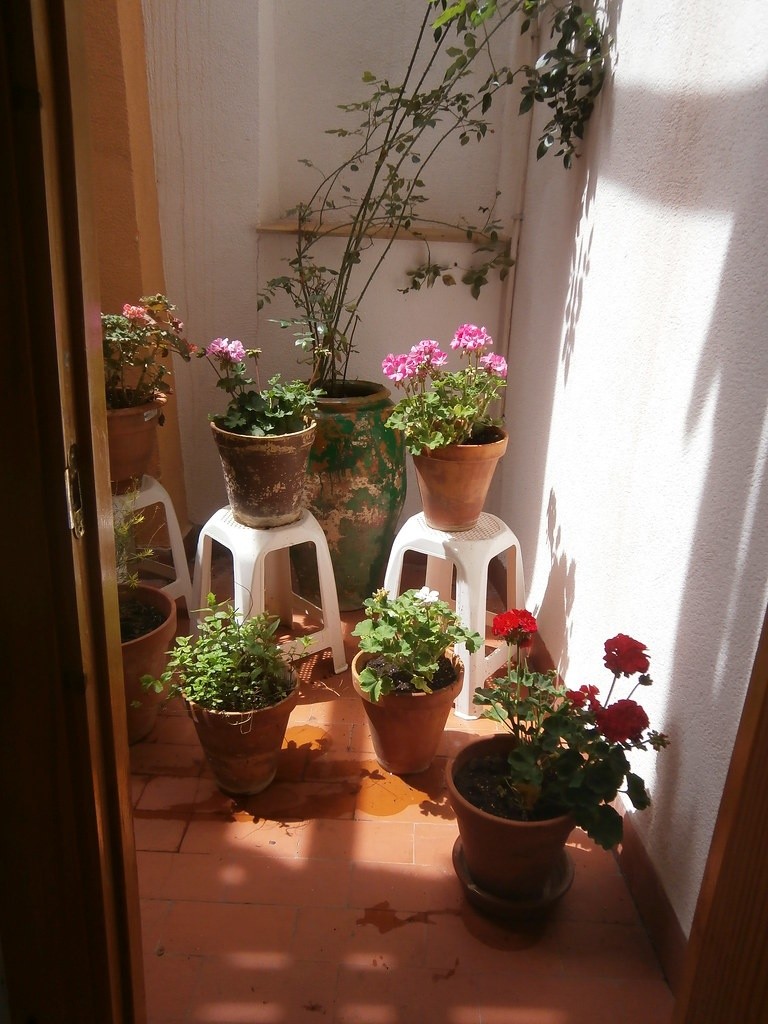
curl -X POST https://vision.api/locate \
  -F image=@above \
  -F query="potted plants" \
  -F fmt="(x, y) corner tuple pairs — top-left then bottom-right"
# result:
(140, 592), (317, 796)
(110, 483), (179, 745)
(258, 1), (626, 606)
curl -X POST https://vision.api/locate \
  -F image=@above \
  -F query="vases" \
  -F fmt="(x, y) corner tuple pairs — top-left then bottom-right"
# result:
(406, 421), (509, 532)
(355, 650), (466, 777)
(105, 389), (169, 488)
(209, 411), (317, 527)
(444, 733), (586, 929)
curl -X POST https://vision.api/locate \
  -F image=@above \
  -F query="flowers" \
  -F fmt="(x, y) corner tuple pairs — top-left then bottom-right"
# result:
(197, 334), (324, 437)
(101, 292), (200, 410)
(352, 588), (485, 700)
(471, 611), (670, 851)
(381, 323), (506, 459)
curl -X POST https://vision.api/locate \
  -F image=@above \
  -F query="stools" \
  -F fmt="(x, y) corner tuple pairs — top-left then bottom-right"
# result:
(385, 510), (528, 722)
(189, 503), (348, 686)
(105, 475), (188, 623)
